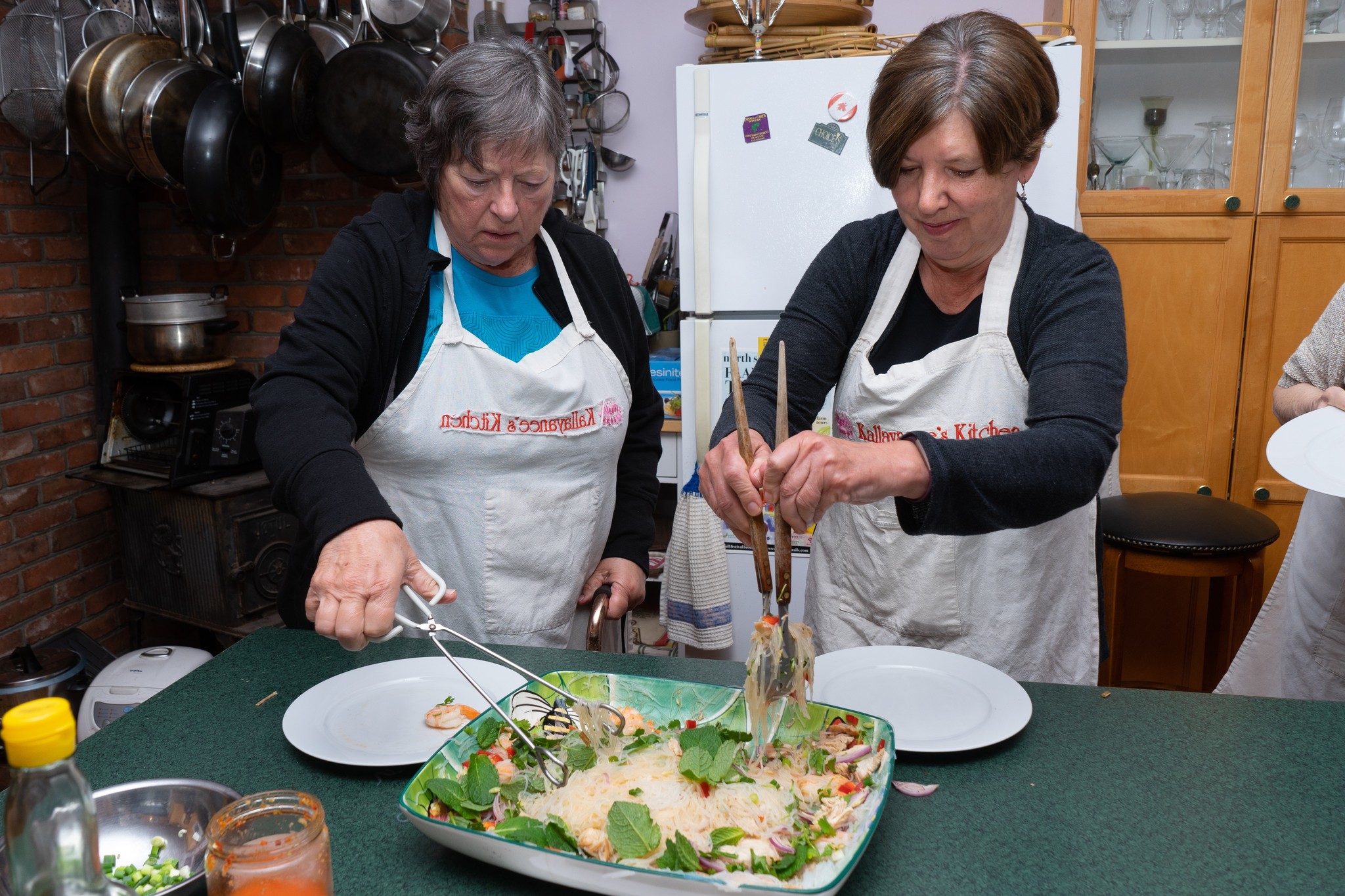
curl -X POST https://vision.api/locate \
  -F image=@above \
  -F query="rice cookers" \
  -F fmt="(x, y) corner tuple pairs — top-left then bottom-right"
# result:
(75, 646), (213, 744)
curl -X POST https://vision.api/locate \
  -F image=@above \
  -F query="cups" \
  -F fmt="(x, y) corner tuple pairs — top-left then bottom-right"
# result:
(548, 37), (565, 72)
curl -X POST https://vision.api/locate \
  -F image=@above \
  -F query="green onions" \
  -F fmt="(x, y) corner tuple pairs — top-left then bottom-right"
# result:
(59, 837), (193, 895)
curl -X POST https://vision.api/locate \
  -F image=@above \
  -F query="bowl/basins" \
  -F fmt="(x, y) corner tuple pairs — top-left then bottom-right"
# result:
(601, 146), (635, 172)
(88, 779), (242, 896)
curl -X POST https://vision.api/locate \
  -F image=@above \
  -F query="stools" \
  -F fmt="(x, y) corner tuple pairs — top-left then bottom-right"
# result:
(1095, 490), (1280, 694)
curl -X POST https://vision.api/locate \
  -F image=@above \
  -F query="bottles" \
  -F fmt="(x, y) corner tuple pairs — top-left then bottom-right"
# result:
(1, 696), (109, 896)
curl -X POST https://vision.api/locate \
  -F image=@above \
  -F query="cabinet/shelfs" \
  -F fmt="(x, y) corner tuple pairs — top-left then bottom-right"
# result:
(1060, 1), (1344, 695)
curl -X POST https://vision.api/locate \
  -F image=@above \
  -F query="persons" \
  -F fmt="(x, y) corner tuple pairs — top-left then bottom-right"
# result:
(251, 36), (662, 655)
(697, 10), (1128, 685)
(1213, 281), (1343, 700)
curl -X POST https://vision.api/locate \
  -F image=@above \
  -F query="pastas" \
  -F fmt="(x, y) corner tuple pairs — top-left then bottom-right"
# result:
(495, 617), (860, 871)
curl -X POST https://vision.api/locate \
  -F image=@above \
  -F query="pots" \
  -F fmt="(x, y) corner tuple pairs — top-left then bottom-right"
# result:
(61, 0), (453, 261)
(0, 645), (87, 719)
(118, 284), (239, 365)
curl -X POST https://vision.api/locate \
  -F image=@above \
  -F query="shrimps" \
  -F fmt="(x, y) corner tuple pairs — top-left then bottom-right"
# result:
(425, 704), (480, 730)
(608, 706), (654, 736)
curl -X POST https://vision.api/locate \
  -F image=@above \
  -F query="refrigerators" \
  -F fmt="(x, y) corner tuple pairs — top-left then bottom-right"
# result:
(677, 44), (1082, 664)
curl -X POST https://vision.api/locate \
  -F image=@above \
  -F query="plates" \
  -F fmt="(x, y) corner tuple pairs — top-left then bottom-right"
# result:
(804, 646), (1033, 751)
(1266, 406), (1345, 498)
(281, 655), (527, 767)
(399, 671), (895, 896)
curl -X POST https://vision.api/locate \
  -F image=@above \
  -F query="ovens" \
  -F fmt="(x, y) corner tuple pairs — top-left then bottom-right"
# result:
(65, 366), (261, 493)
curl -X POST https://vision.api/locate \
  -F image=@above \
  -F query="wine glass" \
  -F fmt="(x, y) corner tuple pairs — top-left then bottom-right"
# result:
(1097, 0), (1341, 42)
(1093, 95), (1345, 188)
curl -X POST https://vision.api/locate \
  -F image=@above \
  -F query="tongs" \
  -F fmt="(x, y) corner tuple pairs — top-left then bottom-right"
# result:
(560, 148), (585, 223)
(365, 559), (625, 787)
(728, 336), (798, 701)
(583, 143), (597, 234)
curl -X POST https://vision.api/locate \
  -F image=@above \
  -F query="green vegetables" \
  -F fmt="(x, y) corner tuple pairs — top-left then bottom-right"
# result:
(425, 717), (879, 884)
